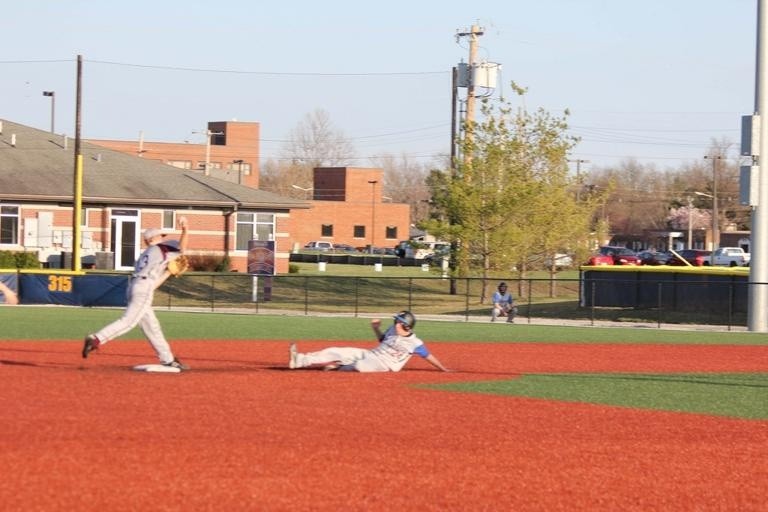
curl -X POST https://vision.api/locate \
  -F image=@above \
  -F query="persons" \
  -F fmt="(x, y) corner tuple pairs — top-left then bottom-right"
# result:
(287, 308), (457, 374)
(488, 280), (519, 323)
(80, 218), (192, 373)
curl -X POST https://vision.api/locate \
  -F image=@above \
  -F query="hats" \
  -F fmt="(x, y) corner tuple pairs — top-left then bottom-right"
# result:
(143, 229), (168, 240)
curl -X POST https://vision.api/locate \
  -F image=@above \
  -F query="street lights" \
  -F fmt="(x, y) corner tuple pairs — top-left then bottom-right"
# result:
(233, 157), (245, 184)
(368, 179), (377, 251)
(43, 90), (56, 132)
(191, 130), (225, 175)
(694, 192), (718, 253)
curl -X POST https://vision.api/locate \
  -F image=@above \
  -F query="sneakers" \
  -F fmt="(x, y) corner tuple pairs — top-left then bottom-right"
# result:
(83, 334), (100, 357)
(288, 343), (297, 369)
(165, 357), (191, 370)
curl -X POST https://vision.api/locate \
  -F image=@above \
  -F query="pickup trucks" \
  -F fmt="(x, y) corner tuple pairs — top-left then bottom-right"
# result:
(302, 241), (339, 254)
(703, 247), (751, 267)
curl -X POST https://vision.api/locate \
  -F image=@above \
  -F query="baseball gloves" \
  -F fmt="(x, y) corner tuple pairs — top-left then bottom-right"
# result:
(168, 255), (190, 277)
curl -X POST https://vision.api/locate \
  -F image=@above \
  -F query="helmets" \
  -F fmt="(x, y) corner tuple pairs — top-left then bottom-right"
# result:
(499, 282), (507, 287)
(393, 311), (414, 330)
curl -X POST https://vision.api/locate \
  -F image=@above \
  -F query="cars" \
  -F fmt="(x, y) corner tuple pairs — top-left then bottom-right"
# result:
(665, 249), (713, 268)
(636, 252), (668, 266)
(515, 244), (573, 272)
(331, 243), (360, 255)
(595, 245), (642, 267)
(424, 248), (451, 264)
(376, 247), (399, 256)
(404, 242), (451, 260)
(352, 245), (382, 254)
(572, 244), (614, 266)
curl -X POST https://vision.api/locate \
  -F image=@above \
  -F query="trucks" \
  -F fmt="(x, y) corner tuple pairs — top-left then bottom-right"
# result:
(394, 235), (461, 258)
